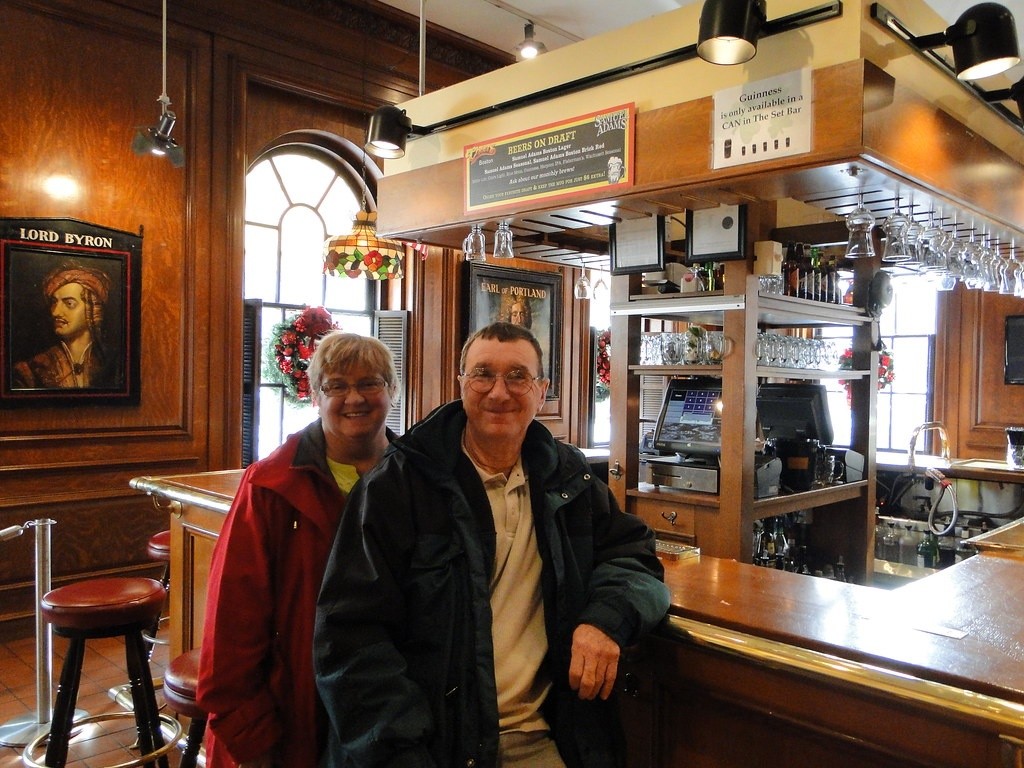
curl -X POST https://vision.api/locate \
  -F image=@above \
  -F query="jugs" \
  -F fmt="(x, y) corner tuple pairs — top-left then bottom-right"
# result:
(1004, 426), (1024, 470)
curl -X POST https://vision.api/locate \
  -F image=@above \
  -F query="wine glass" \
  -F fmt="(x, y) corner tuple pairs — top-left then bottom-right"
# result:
(882, 198), (912, 262)
(895, 206), (925, 267)
(845, 192), (876, 259)
(463, 223), (486, 263)
(493, 221), (514, 258)
(593, 266), (608, 300)
(936, 225), (1024, 299)
(574, 264), (592, 300)
(917, 213), (945, 268)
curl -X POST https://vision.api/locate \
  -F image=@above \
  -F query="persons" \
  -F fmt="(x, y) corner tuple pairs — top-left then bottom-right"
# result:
(314, 323), (670, 768)
(194, 333), (405, 768)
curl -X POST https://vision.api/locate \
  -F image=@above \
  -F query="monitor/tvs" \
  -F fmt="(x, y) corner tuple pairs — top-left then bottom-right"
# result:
(1005, 313), (1024, 384)
(756, 384), (834, 447)
(653, 378), (723, 455)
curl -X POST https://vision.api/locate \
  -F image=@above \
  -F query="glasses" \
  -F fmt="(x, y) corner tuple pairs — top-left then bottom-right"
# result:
(462, 368), (542, 397)
(315, 378), (387, 396)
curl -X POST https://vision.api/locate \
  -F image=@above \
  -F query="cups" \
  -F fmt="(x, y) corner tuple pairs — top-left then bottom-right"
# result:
(680, 267), (705, 292)
(754, 333), (838, 371)
(759, 275), (784, 296)
(640, 332), (725, 365)
(814, 455), (844, 485)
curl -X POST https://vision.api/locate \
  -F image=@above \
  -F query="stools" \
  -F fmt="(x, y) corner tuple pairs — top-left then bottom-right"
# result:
(142, 530), (171, 664)
(163, 647), (211, 768)
(21, 577), (183, 768)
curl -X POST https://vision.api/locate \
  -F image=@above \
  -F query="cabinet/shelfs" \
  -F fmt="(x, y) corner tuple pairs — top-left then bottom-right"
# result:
(611, 222), (880, 580)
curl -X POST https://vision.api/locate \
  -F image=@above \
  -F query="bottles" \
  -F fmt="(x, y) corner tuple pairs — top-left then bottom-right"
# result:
(785, 241), (843, 305)
(753, 518), (846, 583)
(874, 513), (989, 570)
(681, 262), (725, 293)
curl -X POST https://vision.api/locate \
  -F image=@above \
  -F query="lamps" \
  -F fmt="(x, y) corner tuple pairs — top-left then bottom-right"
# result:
(697, 1), (767, 67)
(516, 22), (548, 63)
(321, 68), (405, 281)
(131, 0), (183, 169)
(365, 105), (430, 159)
(914, 2), (1021, 82)
(985, 75), (1024, 128)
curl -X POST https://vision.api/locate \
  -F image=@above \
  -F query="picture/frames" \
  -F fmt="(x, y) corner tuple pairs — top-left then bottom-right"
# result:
(0, 216), (144, 408)
(1004, 315), (1024, 385)
(461, 260), (563, 402)
